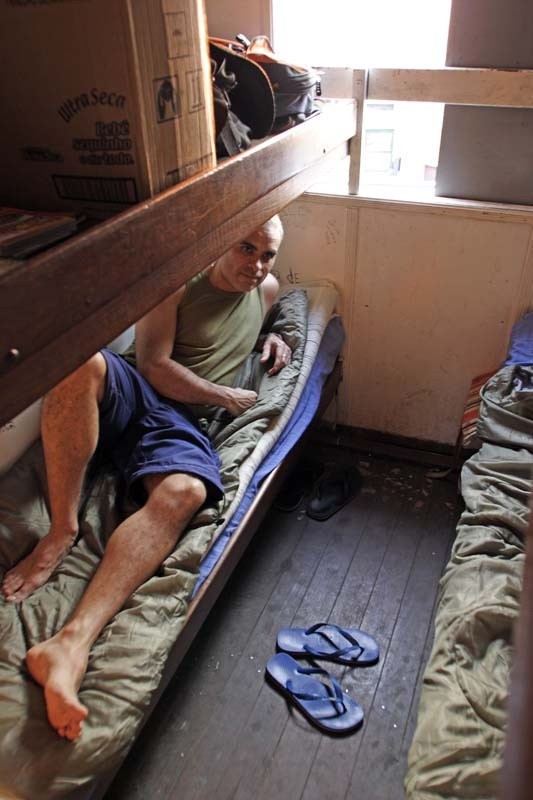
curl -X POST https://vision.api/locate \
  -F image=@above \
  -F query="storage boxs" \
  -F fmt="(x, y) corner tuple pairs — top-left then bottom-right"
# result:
(0, 0), (218, 216)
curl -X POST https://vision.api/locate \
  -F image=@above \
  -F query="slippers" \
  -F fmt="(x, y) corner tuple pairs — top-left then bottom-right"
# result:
(265, 653), (363, 733)
(273, 456), (325, 512)
(276, 623), (379, 664)
(307, 466), (360, 521)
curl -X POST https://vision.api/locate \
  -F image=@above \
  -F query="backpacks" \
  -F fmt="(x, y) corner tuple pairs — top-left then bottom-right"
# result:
(207, 34), (322, 158)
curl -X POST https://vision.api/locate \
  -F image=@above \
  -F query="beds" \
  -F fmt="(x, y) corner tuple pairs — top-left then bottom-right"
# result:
(0, 96), (359, 800)
(400, 305), (533, 800)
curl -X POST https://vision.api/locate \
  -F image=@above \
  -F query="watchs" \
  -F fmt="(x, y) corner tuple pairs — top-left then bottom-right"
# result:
(265, 333), (283, 341)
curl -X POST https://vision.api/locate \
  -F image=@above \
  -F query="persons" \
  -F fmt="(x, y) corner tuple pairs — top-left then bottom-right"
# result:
(2, 213), (292, 740)
